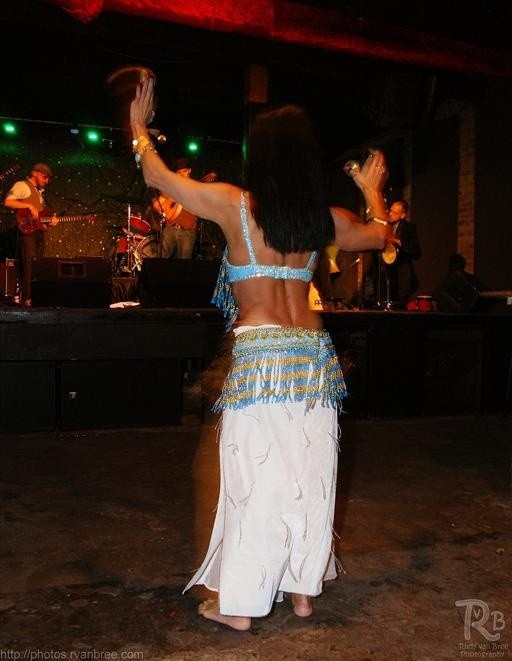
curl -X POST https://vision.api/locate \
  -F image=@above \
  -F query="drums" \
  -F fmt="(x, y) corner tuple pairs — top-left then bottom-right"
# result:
(135, 235), (168, 271)
(116, 236), (140, 254)
(122, 217), (151, 239)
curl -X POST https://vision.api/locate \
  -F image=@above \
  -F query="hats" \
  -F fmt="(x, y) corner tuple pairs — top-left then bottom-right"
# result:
(33, 162), (53, 178)
(172, 157), (192, 170)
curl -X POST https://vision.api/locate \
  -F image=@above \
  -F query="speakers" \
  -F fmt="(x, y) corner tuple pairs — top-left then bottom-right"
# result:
(137, 258), (223, 309)
(31, 257), (112, 308)
(432, 271), (496, 313)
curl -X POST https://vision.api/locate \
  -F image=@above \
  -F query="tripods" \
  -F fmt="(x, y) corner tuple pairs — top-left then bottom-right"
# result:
(112, 207), (143, 279)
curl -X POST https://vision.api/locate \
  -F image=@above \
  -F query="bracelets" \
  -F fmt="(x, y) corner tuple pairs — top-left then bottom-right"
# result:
(367, 214), (390, 227)
(134, 144), (158, 169)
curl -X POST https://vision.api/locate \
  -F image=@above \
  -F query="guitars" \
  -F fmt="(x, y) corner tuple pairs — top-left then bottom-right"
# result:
(17, 208), (96, 234)
(153, 172), (217, 223)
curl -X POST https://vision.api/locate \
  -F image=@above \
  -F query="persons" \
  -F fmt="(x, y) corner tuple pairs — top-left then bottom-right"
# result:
(154, 157), (198, 261)
(133, 76), (392, 632)
(3, 163), (59, 307)
(437, 254), (489, 313)
(379, 198), (421, 308)
